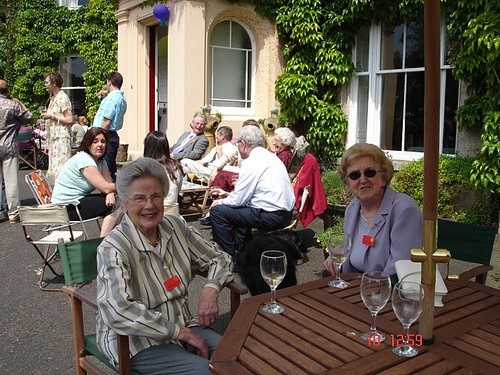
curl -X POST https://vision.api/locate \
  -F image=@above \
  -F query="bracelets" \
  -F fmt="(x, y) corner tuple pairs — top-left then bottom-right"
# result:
(51, 114), (54, 120)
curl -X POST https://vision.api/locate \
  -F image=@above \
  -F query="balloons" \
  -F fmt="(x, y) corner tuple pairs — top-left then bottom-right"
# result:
(153, 4), (170, 21)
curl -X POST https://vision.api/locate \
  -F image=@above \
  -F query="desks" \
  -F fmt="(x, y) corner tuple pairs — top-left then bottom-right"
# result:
(209, 273), (500, 375)
(178, 182), (210, 219)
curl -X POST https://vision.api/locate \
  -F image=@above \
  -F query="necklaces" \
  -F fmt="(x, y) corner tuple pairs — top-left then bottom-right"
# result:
(360, 209), (375, 228)
(145, 231), (159, 247)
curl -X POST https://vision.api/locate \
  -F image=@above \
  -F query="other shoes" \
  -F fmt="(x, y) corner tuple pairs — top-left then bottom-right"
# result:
(198, 217), (211, 225)
(10, 219), (20, 224)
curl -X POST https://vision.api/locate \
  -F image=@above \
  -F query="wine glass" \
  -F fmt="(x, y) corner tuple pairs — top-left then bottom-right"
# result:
(183, 170), (211, 188)
(392, 281), (425, 357)
(260, 250), (287, 314)
(326, 233), (352, 289)
(97, 85), (108, 98)
(359, 271), (391, 343)
(40, 106), (47, 122)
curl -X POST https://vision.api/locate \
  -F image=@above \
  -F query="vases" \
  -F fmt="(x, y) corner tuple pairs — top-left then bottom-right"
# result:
(116, 144), (128, 162)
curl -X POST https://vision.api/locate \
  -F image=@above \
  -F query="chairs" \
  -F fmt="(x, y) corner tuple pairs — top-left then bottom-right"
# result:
(239, 148), (326, 265)
(59, 238), (248, 375)
(437, 218), (498, 286)
(25, 169), (101, 261)
(15, 131), (36, 170)
(16, 206), (85, 291)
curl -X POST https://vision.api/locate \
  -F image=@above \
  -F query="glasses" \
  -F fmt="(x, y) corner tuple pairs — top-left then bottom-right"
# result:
(125, 193), (164, 205)
(347, 168), (385, 180)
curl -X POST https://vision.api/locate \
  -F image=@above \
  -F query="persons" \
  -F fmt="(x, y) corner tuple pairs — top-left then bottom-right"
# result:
(144, 130), (181, 216)
(210, 125), (296, 263)
(51, 127), (125, 237)
(94, 71), (127, 183)
(96, 158), (235, 375)
(0, 80), (35, 224)
(324, 144), (422, 277)
(70, 114), (89, 148)
(18, 119), (47, 170)
(42, 73), (74, 191)
(169, 110), (298, 224)
